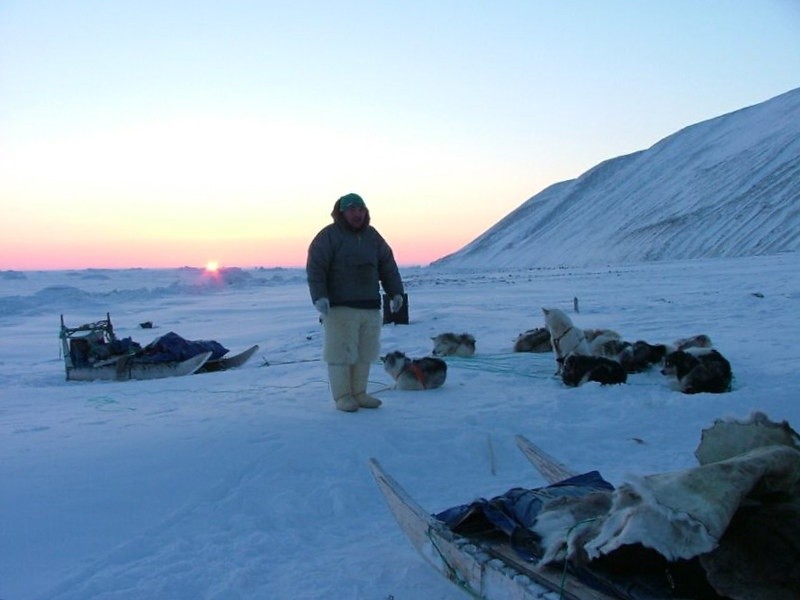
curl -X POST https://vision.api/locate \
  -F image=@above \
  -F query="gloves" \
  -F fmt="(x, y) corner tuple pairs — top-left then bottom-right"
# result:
(316, 298), (329, 315)
(392, 294), (403, 314)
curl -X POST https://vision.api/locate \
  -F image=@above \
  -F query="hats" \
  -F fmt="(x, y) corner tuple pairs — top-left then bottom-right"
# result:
(340, 193), (364, 212)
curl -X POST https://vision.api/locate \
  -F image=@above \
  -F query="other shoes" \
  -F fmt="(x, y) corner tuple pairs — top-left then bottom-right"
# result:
(336, 395), (358, 411)
(356, 393), (381, 408)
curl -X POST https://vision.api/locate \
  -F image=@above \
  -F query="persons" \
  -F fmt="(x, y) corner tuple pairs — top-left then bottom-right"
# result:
(307, 193), (403, 413)
